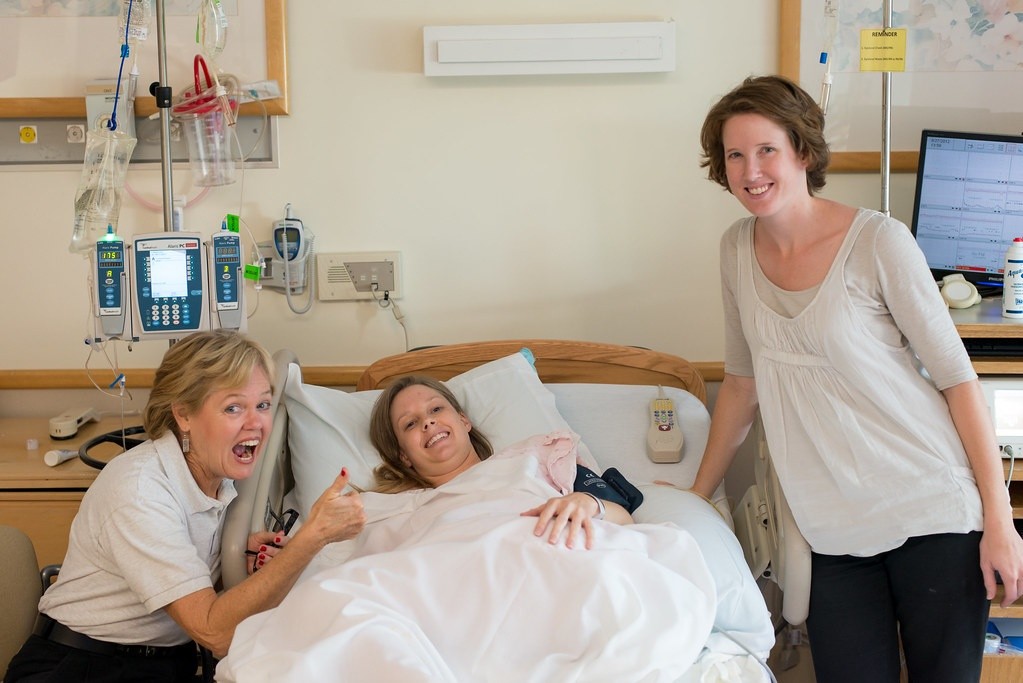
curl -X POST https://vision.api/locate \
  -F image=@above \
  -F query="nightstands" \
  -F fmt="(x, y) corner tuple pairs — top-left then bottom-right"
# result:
(0, 415), (147, 586)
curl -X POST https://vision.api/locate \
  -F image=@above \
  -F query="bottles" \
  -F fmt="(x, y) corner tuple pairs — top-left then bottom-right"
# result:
(1002, 237), (1023, 319)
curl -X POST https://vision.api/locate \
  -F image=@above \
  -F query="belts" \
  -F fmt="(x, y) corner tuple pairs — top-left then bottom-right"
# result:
(32, 614), (195, 658)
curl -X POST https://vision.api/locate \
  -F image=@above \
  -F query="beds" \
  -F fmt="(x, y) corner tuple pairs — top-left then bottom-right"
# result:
(214, 340), (811, 683)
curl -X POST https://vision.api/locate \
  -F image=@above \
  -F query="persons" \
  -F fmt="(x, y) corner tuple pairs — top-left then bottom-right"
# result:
(654, 74), (1023, 683)
(2, 329), (365, 683)
(346, 373), (635, 550)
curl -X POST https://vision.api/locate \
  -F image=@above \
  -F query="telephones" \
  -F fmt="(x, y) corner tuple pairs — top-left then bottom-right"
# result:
(271, 201), (307, 294)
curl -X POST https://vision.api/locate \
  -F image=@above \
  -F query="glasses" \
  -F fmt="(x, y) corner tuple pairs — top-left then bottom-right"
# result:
(245, 509), (299, 572)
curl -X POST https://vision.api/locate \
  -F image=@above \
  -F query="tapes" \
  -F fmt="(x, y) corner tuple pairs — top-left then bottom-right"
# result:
(984, 632), (1001, 652)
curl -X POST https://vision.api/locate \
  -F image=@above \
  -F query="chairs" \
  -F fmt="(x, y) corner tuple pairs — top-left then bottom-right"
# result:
(0, 525), (61, 683)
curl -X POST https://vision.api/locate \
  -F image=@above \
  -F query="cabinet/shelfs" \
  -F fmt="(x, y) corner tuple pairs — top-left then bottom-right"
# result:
(943, 297), (1023, 683)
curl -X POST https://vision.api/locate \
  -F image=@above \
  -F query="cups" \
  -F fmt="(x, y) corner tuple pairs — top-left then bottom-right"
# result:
(170, 93), (236, 187)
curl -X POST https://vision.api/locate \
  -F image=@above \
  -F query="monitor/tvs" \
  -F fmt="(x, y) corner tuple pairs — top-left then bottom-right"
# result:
(911, 129), (1023, 287)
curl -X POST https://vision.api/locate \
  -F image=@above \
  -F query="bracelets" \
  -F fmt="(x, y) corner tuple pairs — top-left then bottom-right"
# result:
(584, 491), (605, 520)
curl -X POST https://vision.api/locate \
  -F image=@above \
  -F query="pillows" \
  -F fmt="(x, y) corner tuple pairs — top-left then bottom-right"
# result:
(284, 347), (604, 525)
